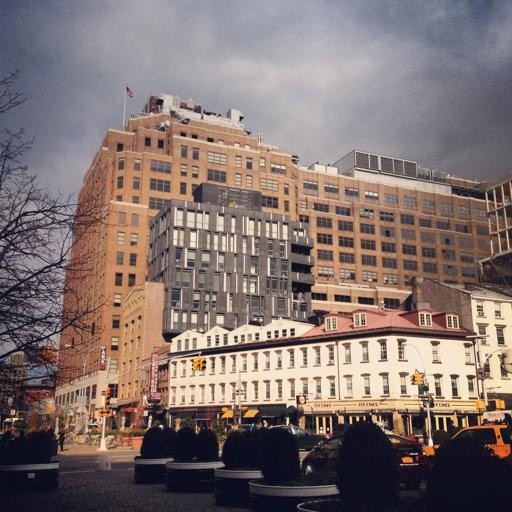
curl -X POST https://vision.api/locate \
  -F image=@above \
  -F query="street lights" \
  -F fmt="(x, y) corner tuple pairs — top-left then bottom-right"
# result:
(64, 343), (107, 436)
(475, 334), (510, 411)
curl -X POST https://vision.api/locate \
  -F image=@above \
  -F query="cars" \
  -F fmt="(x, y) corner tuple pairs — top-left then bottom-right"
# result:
(1, 412), (99, 441)
(229, 419), (512, 495)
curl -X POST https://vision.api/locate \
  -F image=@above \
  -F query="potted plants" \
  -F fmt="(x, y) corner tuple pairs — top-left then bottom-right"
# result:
(1, 430), (58, 491)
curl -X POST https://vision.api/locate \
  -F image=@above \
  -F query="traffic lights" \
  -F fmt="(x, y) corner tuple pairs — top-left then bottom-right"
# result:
(104, 388), (110, 409)
(418, 374), (425, 385)
(412, 374), (419, 385)
(199, 357), (207, 372)
(196, 358), (201, 367)
(46, 346), (57, 363)
(191, 358), (197, 371)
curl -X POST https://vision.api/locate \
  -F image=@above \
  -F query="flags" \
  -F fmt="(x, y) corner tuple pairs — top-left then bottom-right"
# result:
(127, 86), (133, 99)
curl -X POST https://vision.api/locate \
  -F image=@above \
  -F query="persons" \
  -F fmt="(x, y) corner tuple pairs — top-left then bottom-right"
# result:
(58, 426), (66, 451)
